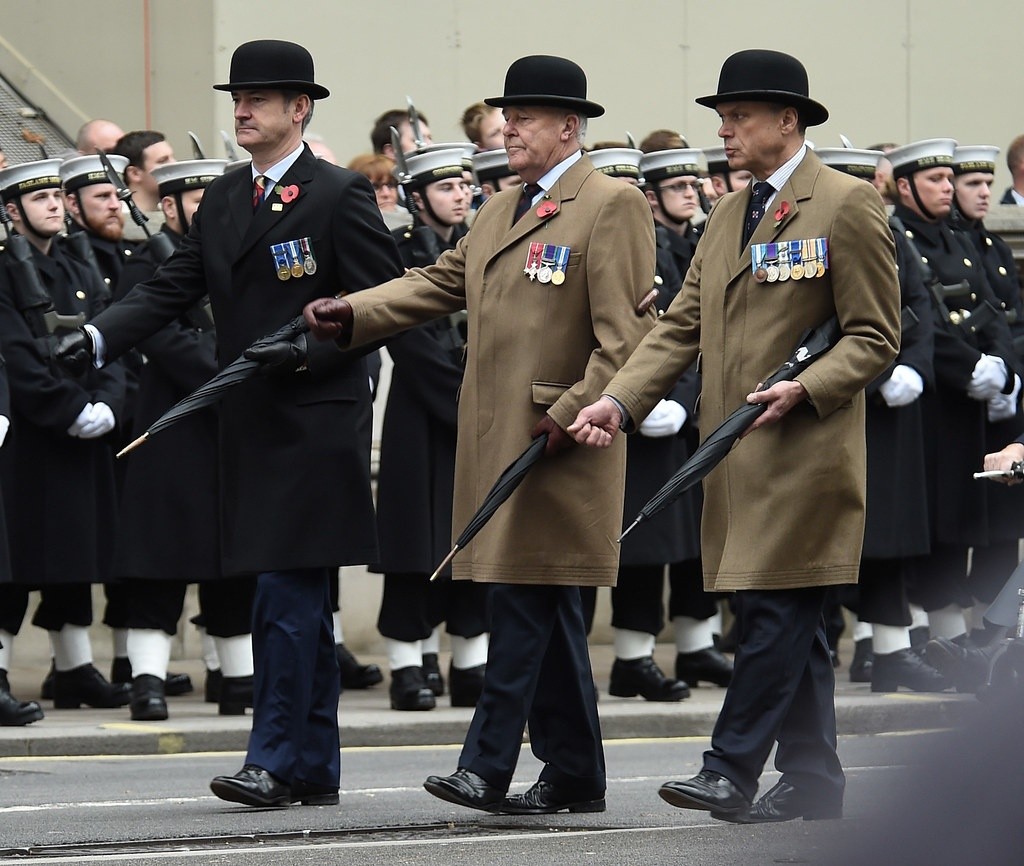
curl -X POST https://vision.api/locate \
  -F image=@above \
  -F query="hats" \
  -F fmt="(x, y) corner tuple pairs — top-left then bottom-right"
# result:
(813, 138), (1000, 178)
(0, 147), (131, 205)
(695, 50), (828, 128)
(148, 157), (252, 196)
(213, 40), (330, 101)
(391, 142), (520, 202)
(586, 143), (746, 183)
(484, 54), (607, 118)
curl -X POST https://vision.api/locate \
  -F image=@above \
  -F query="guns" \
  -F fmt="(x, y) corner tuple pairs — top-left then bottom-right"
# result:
(383, 124), (462, 366)
(96, 144), (216, 332)
(1, 196), (88, 332)
(841, 133), (1001, 341)
(678, 135), (712, 213)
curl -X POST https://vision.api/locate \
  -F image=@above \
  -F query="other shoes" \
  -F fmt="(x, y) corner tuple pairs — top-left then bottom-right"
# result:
(205, 669), (222, 704)
(423, 651), (445, 696)
(592, 680), (599, 700)
(336, 643), (383, 688)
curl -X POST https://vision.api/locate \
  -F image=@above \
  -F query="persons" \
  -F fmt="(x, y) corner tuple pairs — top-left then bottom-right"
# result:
(572, 51), (903, 829)
(0, 115), (1024, 727)
(294, 54), (658, 815)
(49, 40), (403, 805)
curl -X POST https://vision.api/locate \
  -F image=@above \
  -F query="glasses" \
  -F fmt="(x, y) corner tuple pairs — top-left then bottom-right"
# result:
(660, 183), (700, 192)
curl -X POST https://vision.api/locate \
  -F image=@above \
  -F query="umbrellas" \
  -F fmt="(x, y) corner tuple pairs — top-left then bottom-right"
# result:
(430, 288), (660, 583)
(112, 290), (344, 459)
(617, 315), (841, 544)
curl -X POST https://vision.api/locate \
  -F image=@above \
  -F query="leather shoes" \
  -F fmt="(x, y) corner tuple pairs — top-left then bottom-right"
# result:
(449, 662), (486, 706)
(423, 767), (506, 814)
(850, 624), (1015, 691)
(675, 645), (734, 687)
(658, 769), (750, 823)
(210, 763), (291, 808)
(290, 781), (340, 805)
(0, 657), (193, 727)
(499, 779), (606, 814)
(737, 780), (843, 823)
(391, 665), (436, 711)
(219, 675), (255, 714)
(608, 655), (690, 700)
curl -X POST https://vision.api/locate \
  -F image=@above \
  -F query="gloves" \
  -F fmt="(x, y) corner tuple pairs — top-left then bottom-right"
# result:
(987, 373), (1021, 422)
(967, 352), (1007, 401)
(531, 415), (580, 464)
(0, 415), (10, 448)
(244, 335), (305, 373)
(51, 329), (91, 369)
(640, 397), (689, 439)
(879, 364), (923, 407)
(303, 297), (350, 345)
(68, 401), (116, 438)
